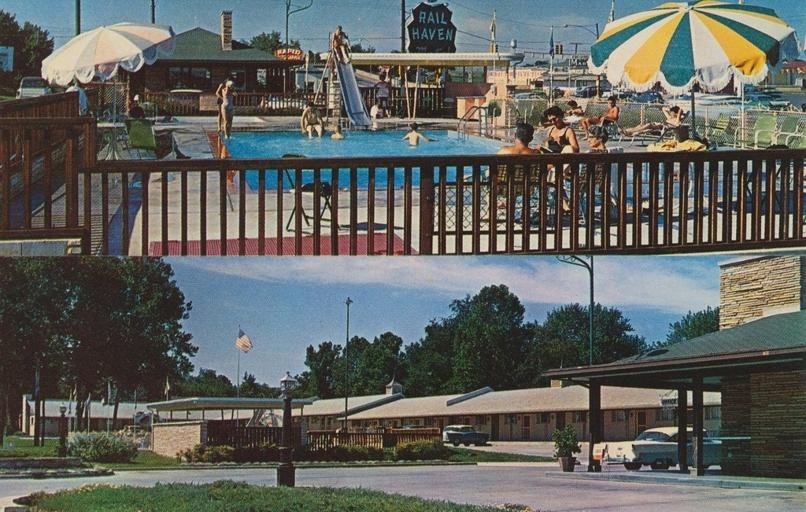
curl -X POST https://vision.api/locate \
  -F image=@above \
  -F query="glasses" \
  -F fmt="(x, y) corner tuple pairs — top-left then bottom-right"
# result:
(548, 116), (558, 122)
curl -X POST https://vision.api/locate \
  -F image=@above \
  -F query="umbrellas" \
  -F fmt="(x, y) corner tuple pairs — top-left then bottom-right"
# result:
(41, 23), (176, 144)
(586, 0), (801, 140)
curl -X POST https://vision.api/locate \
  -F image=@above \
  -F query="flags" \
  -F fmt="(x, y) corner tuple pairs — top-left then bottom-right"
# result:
(490, 11), (496, 42)
(235, 327), (253, 353)
(549, 28), (554, 59)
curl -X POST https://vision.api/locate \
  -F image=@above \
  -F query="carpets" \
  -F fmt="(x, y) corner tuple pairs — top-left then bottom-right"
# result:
(146, 231), (420, 257)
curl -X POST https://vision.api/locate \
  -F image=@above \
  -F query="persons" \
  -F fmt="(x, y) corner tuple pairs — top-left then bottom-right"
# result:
(375, 75), (391, 111)
(331, 125), (345, 140)
(65, 83), (89, 117)
(215, 75), (236, 133)
(130, 107), (191, 159)
(222, 80), (238, 140)
(331, 25), (351, 63)
(484, 96), (619, 218)
(401, 123), (429, 146)
(300, 102), (322, 139)
(617, 106), (683, 136)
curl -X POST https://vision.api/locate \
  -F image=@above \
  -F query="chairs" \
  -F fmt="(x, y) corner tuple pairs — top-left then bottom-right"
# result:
(280, 153), (340, 232)
(479, 95), (805, 232)
(122, 117), (179, 180)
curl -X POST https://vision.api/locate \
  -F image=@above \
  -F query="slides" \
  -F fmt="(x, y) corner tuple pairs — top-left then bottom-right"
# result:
(333, 49), (372, 127)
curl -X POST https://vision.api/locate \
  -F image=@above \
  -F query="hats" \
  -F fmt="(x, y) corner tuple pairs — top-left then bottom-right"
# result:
(589, 124), (609, 142)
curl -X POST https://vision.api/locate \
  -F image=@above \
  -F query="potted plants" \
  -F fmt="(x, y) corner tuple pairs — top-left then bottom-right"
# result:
(551, 421), (583, 473)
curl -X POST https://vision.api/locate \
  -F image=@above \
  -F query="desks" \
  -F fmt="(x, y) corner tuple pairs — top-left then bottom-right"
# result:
(95, 121), (127, 160)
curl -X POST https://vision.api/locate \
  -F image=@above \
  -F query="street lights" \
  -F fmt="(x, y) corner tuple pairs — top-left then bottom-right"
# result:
(344, 296), (354, 433)
(510, 39), (518, 84)
(562, 23), (601, 97)
(274, 369), (299, 488)
(57, 401), (69, 457)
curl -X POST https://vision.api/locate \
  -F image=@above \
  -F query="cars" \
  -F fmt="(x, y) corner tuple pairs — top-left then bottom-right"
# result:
(259, 97), (308, 110)
(441, 422), (490, 444)
(677, 85), (792, 111)
(14, 75), (53, 99)
(402, 424), (426, 429)
(604, 424), (714, 472)
(511, 86), (663, 108)
(162, 89), (204, 114)
